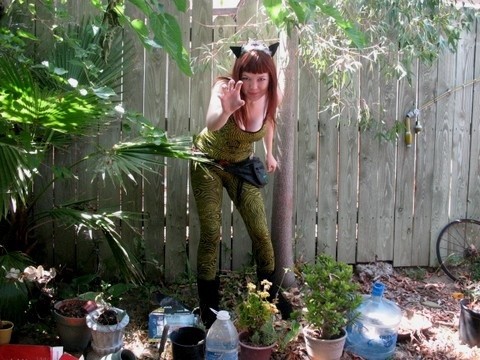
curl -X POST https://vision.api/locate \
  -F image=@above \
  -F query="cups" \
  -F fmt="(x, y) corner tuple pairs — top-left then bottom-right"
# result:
(170, 327), (206, 360)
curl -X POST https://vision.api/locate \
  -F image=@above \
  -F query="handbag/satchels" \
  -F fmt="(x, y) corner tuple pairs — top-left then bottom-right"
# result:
(228, 158), (268, 188)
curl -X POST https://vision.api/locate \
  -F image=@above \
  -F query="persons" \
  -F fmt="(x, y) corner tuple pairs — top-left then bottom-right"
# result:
(187, 42), (288, 329)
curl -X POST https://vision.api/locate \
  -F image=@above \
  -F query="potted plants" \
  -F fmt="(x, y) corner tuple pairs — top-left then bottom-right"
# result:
(299, 252), (362, 360)
(449, 255), (480, 348)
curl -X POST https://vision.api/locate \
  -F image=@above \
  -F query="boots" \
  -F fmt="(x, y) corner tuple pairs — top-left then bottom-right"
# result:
(256, 269), (294, 319)
(196, 276), (221, 331)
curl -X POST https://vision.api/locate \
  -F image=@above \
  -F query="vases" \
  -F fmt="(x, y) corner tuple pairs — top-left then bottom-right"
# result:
(86, 307), (130, 356)
(54, 299), (93, 351)
(0, 321), (14, 344)
(238, 331), (277, 360)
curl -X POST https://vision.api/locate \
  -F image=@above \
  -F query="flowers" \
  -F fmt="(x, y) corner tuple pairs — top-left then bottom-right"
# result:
(235, 279), (280, 346)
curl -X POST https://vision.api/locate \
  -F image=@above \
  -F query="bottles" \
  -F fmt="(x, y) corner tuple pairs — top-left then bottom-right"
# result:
(206, 308), (239, 360)
(345, 282), (401, 360)
